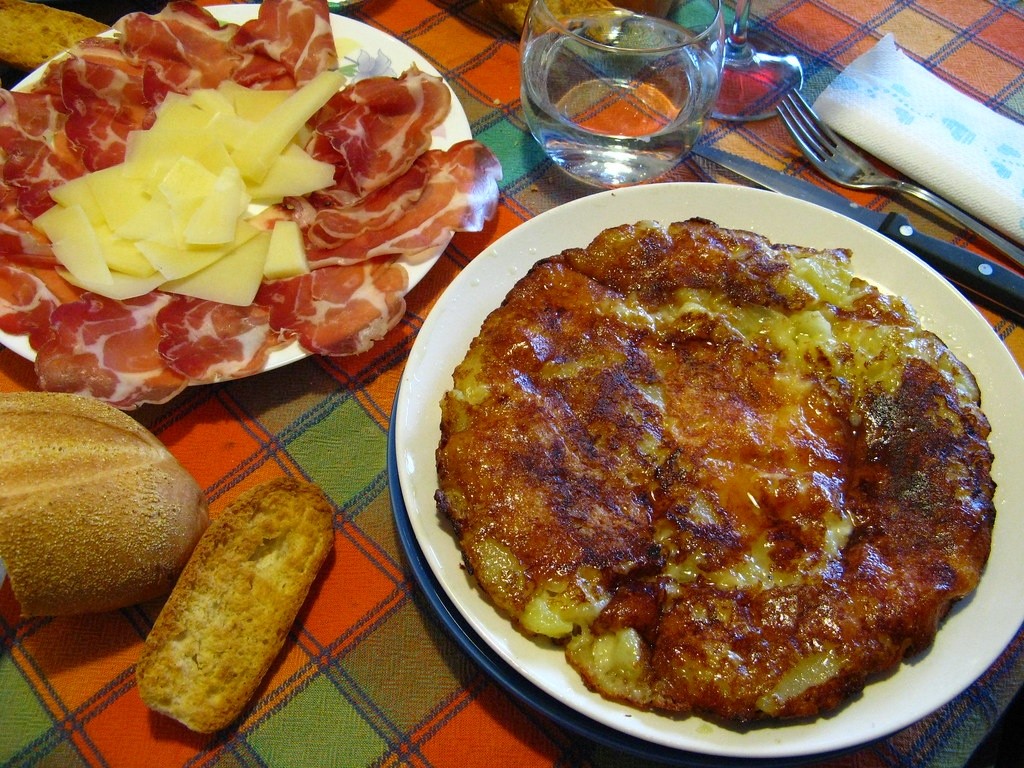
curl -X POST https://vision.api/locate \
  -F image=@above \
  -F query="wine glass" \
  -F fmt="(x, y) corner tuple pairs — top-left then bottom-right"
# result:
(706, 0), (805, 121)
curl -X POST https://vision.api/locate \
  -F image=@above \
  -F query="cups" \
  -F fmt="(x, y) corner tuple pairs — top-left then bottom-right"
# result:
(518, 0), (726, 188)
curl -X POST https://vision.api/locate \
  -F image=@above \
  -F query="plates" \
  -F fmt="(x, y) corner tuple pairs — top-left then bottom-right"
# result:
(386, 373), (752, 768)
(0, 5), (474, 382)
(393, 182), (1024, 757)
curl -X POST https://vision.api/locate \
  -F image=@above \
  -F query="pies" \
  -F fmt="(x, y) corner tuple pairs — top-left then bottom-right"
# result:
(438, 218), (1000, 726)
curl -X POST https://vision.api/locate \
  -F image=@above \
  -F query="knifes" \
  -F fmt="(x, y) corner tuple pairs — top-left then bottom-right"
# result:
(691, 145), (1024, 319)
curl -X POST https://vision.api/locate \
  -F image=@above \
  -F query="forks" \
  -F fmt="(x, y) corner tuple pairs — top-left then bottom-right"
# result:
(775, 87), (1024, 268)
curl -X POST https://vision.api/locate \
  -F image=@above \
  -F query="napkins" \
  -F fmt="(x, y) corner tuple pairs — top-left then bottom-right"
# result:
(811, 32), (1024, 246)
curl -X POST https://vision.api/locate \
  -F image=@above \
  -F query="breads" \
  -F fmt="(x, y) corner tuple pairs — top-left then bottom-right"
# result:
(0, 392), (210, 618)
(478, 0), (626, 44)
(136, 475), (338, 734)
(0, 0), (118, 71)
